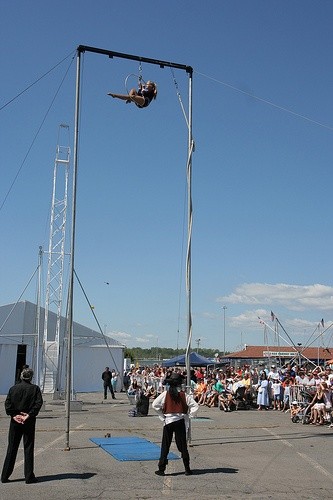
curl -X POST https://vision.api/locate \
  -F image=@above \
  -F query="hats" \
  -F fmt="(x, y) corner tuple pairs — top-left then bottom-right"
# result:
(313, 370), (319, 374)
(219, 369), (223, 372)
(292, 401), (297, 404)
(272, 365), (276, 368)
(165, 371), (185, 384)
(20, 369), (34, 380)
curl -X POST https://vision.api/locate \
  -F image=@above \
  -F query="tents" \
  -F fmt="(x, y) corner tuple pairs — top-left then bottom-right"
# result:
(162, 351), (220, 370)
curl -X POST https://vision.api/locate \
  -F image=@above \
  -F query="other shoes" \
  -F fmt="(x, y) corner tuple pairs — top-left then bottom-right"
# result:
(185, 468), (191, 475)
(155, 469), (165, 476)
(197, 401), (333, 427)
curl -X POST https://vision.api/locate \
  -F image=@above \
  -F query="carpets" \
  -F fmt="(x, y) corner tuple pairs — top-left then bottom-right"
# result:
(89, 437), (181, 461)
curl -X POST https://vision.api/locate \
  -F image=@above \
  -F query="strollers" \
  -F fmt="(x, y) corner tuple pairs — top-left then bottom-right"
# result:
(291, 387), (317, 424)
(232, 383), (250, 410)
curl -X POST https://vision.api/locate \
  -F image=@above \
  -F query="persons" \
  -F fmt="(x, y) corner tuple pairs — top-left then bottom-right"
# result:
(107, 81), (157, 108)
(23, 365), (29, 369)
(123, 363), (333, 426)
(102, 367), (116, 399)
(111, 369), (117, 392)
(152, 376), (199, 475)
(1, 368), (43, 484)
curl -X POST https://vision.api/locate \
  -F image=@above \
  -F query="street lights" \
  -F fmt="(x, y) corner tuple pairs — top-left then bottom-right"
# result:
(195, 339), (200, 348)
(221, 305), (228, 356)
